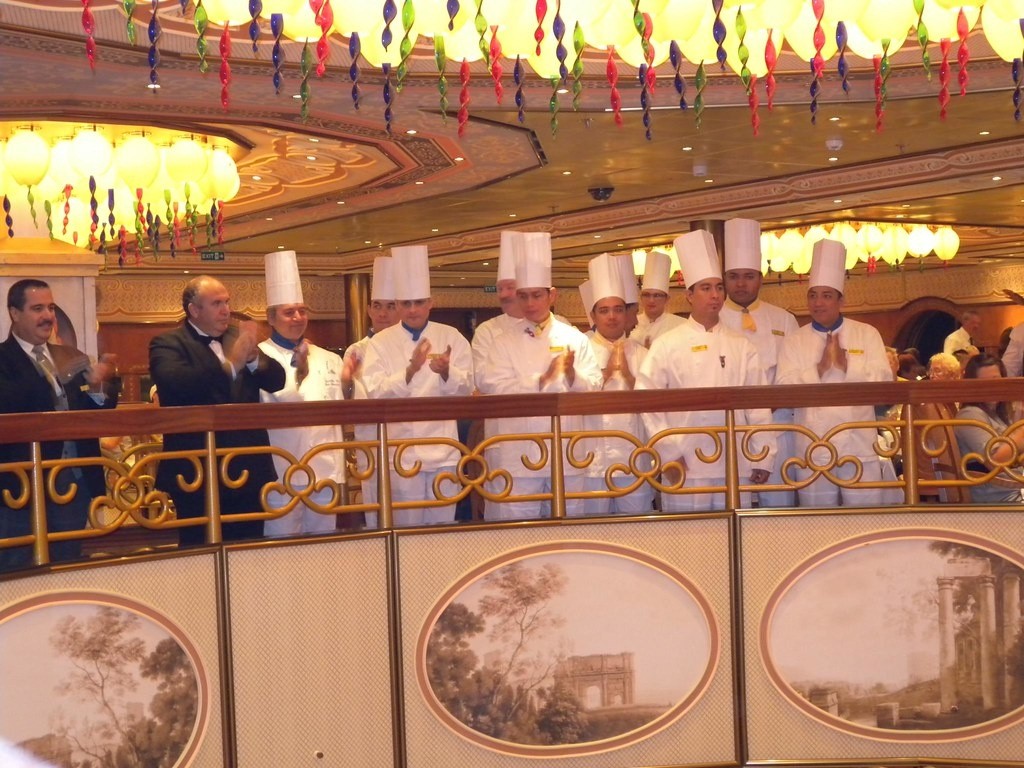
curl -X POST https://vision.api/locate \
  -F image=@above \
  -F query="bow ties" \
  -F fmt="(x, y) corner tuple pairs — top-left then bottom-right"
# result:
(198, 336), (224, 345)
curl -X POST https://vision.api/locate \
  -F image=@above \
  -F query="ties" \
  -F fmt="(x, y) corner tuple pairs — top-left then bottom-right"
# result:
(969, 336), (973, 345)
(31, 345), (63, 390)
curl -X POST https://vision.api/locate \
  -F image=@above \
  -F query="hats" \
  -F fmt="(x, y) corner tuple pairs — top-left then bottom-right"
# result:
(616, 255), (639, 304)
(370, 256), (395, 301)
(724, 218), (761, 272)
(642, 251), (671, 294)
(392, 245), (431, 300)
(673, 229), (722, 289)
(517, 232), (552, 289)
(264, 250), (304, 306)
(588, 253), (625, 311)
(808, 238), (846, 295)
(497, 230), (517, 280)
(579, 280), (595, 328)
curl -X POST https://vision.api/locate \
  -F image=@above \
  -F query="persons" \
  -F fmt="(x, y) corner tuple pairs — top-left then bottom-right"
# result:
(775, 237), (895, 508)
(577, 254), (656, 515)
(480, 233), (604, 523)
(632, 228), (778, 511)
(0, 278), (122, 577)
(578, 252), (690, 346)
(879, 309), (1024, 504)
(717, 216), (799, 506)
(343, 254), (402, 380)
(472, 232), (571, 386)
(244, 249), (368, 541)
(146, 273), (286, 551)
(363, 245), (476, 530)
